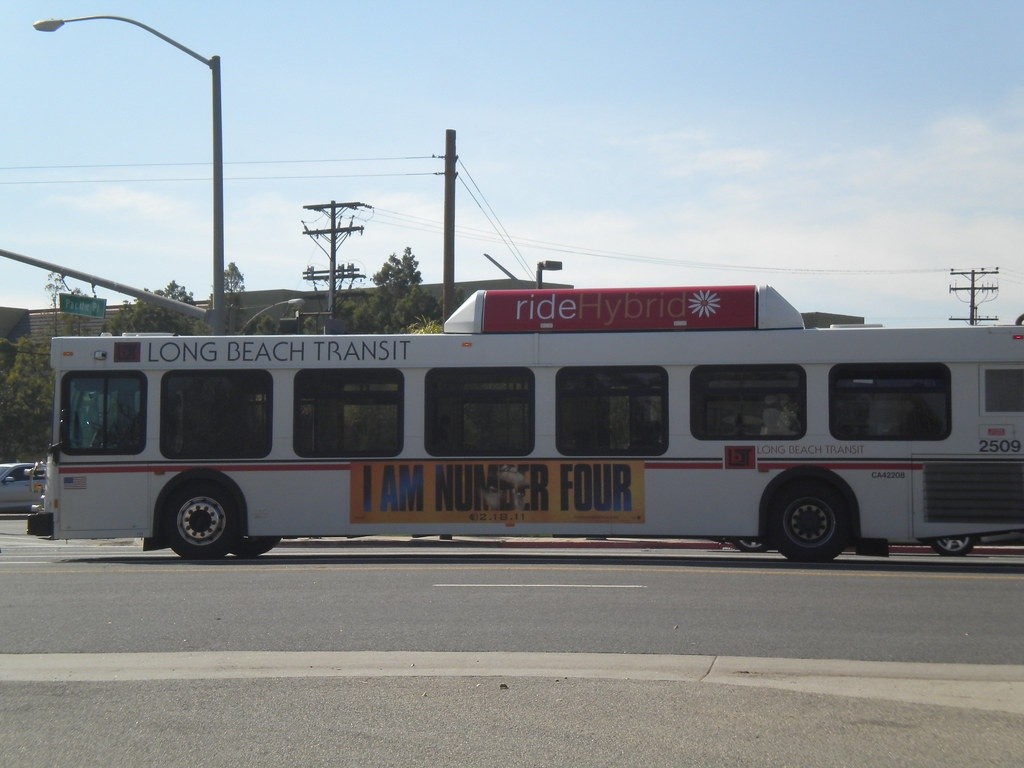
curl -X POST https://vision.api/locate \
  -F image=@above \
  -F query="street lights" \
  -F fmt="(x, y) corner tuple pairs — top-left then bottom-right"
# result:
(1, 14), (226, 336)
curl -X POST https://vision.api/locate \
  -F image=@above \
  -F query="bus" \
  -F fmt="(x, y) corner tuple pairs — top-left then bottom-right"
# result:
(24, 284), (1023, 562)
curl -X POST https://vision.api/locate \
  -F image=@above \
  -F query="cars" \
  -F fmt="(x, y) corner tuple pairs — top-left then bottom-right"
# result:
(0, 462), (47, 513)
